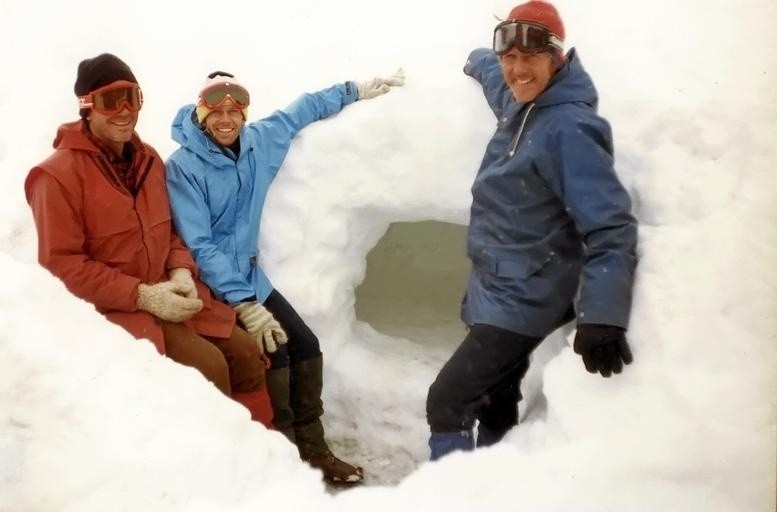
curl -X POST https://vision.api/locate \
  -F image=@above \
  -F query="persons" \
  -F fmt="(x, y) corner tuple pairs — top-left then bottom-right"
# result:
(423, 0), (640, 460)
(24, 52), (277, 432)
(162, 67), (407, 486)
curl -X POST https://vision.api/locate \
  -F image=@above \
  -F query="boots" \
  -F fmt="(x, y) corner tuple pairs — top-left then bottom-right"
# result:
(289, 352), (366, 486)
(230, 364), (296, 450)
(428, 429), (476, 461)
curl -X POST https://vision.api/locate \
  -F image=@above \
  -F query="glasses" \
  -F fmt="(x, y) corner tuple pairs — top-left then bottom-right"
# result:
(197, 81), (251, 109)
(493, 18), (565, 55)
(77, 80), (145, 116)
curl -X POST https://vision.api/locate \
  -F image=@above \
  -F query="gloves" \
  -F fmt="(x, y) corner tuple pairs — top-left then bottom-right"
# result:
(137, 265), (205, 323)
(573, 323), (634, 379)
(354, 67), (408, 102)
(232, 300), (288, 357)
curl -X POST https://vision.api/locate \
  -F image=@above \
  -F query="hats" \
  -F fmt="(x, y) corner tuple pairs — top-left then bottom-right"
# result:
(72, 51), (139, 120)
(194, 71), (249, 128)
(500, 0), (565, 64)
